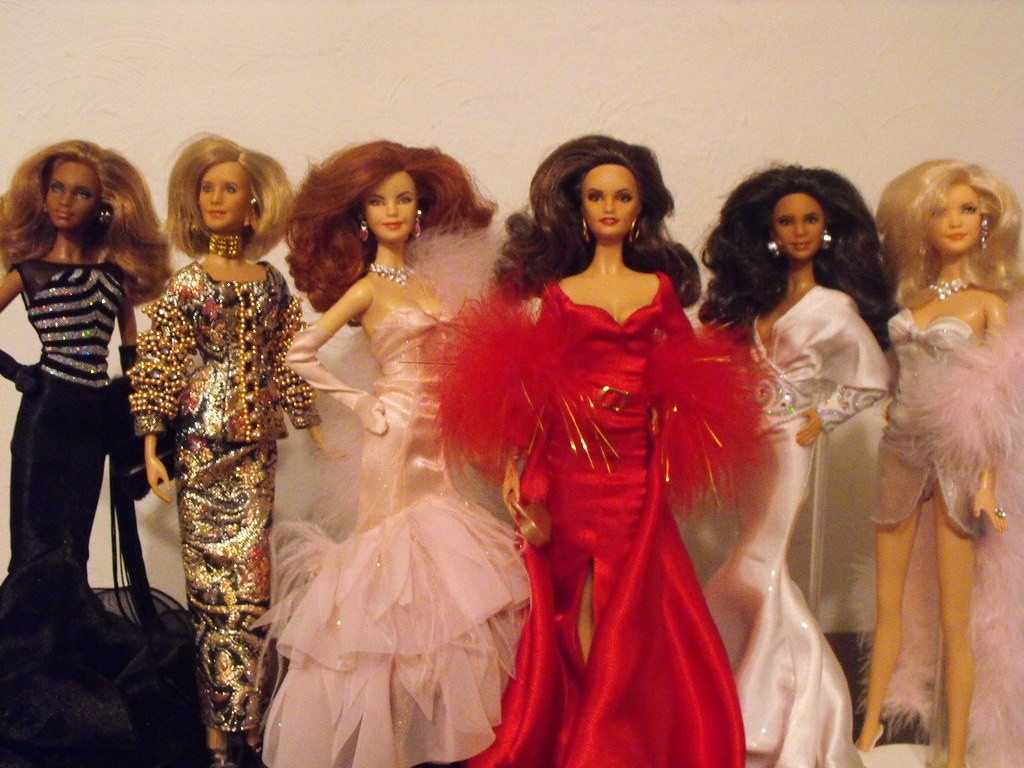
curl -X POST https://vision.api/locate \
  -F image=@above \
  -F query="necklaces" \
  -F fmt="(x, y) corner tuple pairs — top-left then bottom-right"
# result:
(368, 262), (416, 286)
(928, 278), (973, 301)
(207, 232), (243, 258)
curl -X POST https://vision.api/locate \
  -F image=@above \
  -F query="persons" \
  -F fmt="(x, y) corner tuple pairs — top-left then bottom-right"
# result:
(0, 135), (1024, 768)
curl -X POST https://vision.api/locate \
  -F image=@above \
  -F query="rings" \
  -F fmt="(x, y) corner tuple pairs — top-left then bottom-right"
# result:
(995, 507), (1008, 518)
